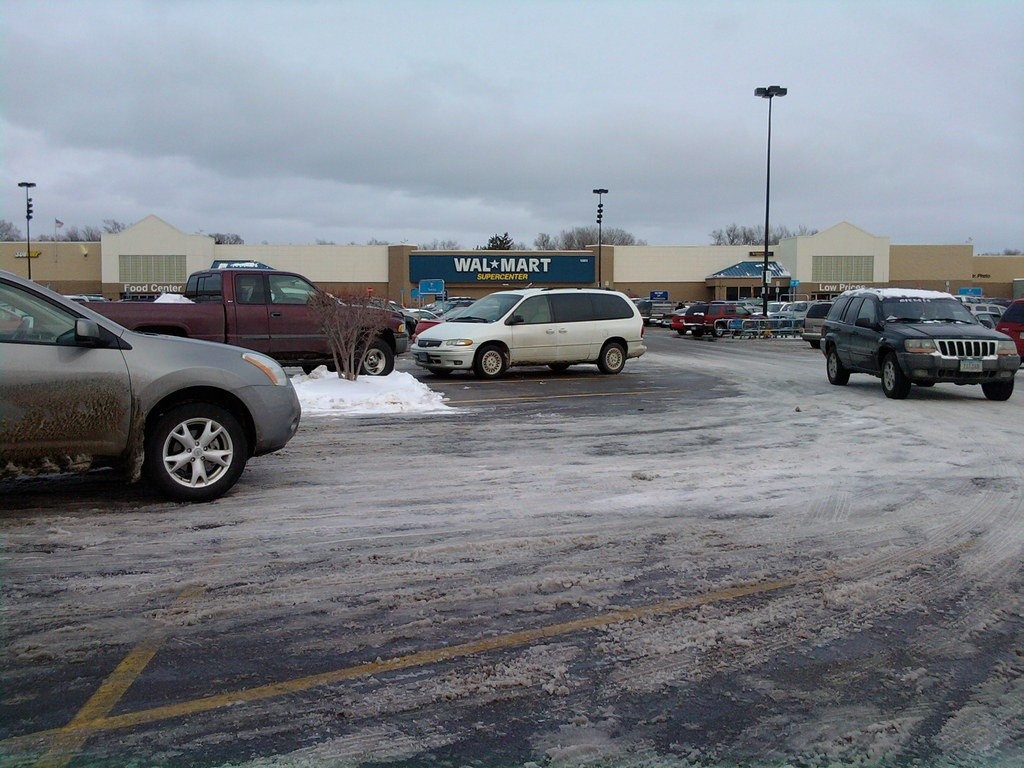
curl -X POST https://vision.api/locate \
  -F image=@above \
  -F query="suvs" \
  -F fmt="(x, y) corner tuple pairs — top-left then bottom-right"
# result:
(820, 289), (1021, 402)
(0, 269), (302, 502)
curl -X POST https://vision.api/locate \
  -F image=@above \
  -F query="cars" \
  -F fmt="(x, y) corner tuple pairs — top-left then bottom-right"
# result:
(631, 296), (1024, 369)
(0, 296), (477, 343)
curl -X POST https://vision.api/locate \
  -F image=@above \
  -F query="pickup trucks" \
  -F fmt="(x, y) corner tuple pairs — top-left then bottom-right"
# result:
(77, 269), (408, 376)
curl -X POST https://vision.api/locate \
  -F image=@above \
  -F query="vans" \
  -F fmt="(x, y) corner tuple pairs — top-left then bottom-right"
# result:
(411, 287), (647, 380)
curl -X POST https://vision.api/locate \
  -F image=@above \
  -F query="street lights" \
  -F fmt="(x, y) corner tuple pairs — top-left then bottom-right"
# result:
(754, 85), (787, 319)
(19, 182), (37, 281)
(593, 189), (608, 290)
